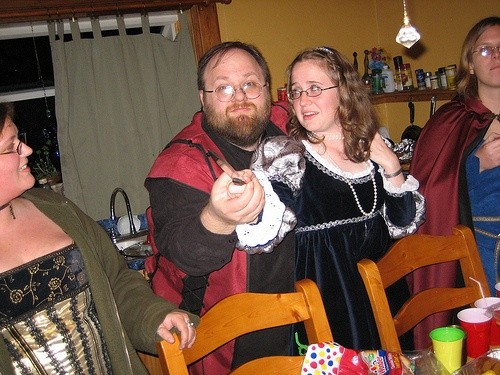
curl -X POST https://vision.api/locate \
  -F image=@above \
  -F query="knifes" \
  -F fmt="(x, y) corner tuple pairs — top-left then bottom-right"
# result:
(207, 150), (245, 185)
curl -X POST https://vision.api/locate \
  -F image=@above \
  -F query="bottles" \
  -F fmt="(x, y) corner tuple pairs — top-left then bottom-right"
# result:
(352, 50), (457, 94)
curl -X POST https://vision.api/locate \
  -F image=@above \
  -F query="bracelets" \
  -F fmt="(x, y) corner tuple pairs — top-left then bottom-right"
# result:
(384, 166), (403, 179)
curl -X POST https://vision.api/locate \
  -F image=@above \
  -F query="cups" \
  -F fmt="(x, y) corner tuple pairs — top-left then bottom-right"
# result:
(474, 297), (500, 345)
(495, 282), (500, 297)
(429, 327), (465, 374)
(457, 308), (493, 358)
(278, 87), (288, 101)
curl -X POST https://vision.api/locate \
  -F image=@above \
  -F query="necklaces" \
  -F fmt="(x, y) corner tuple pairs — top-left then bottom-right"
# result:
(306, 131), (379, 216)
(0, 203), (16, 219)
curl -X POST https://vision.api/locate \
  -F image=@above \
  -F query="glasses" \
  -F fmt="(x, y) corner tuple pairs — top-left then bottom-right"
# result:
(203, 81), (270, 102)
(0, 133), (26, 156)
(473, 46), (500, 57)
(286, 84), (338, 100)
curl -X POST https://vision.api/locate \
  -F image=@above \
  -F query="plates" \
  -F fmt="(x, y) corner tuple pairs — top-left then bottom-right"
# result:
(452, 348), (500, 375)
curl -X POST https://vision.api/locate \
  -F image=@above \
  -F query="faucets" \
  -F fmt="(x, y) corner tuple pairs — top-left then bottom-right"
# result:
(109, 188), (136, 234)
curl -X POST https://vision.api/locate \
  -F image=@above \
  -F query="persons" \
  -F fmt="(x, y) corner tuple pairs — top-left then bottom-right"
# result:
(409, 17), (500, 354)
(142, 40), (305, 375)
(0, 103), (196, 375)
(226, 46), (425, 357)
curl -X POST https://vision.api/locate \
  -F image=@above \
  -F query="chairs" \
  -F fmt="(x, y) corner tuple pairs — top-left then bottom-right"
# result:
(357, 225), (492, 354)
(155, 278), (334, 375)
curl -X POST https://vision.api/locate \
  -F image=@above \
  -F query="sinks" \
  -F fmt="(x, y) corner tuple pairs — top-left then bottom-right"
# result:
(114, 239), (144, 263)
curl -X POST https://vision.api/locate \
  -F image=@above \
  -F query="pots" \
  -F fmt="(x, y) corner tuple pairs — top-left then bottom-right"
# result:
(401, 102), (422, 142)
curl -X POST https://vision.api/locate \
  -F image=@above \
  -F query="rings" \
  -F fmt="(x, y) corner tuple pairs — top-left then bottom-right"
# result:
(188, 323), (195, 327)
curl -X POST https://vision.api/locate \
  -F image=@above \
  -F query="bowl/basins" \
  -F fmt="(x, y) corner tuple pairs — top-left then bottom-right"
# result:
(117, 216), (141, 235)
